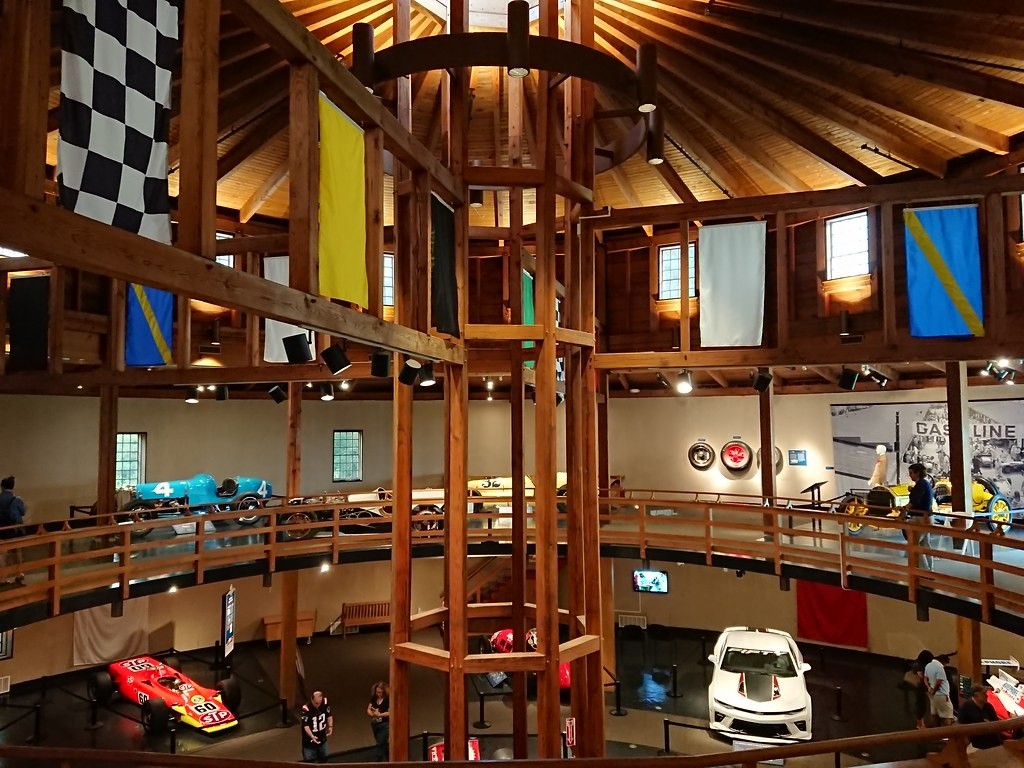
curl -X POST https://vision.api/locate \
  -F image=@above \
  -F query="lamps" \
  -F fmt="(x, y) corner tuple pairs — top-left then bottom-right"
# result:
(839, 360), (893, 393)
(749, 371), (775, 393)
(369, 349), (391, 376)
(530, 388), (565, 409)
(317, 381), (339, 403)
(840, 309), (850, 336)
(185, 388), (199, 406)
(281, 330), (314, 366)
(216, 385), (229, 404)
(208, 319), (223, 345)
(981, 357), (1018, 383)
(267, 384), (285, 403)
(345, 2), (664, 208)
(419, 361), (437, 386)
(676, 370), (694, 393)
(397, 356), (419, 385)
(322, 338), (352, 373)
(672, 326), (679, 349)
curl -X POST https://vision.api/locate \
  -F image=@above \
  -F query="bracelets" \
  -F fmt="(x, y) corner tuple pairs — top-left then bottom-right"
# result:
(328, 726), (332, 729)
(311, 736), (314, 739)
(903, 506), (906, 509)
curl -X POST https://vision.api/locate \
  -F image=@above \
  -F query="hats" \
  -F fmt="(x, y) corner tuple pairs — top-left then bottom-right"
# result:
(970, 683), (991, 697)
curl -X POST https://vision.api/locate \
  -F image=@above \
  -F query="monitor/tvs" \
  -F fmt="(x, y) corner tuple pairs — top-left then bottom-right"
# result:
(632, 570), (669, 594)
(485, 672), (507, 688)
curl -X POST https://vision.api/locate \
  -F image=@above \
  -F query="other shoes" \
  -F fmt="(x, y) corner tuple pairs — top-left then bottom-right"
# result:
(0, 580), (7, 586)
(15, 575), (24, 583)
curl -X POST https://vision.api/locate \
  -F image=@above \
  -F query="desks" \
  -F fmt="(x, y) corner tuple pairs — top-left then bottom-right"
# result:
(261, 612), (314, 648)
(469, 675), (513, 729)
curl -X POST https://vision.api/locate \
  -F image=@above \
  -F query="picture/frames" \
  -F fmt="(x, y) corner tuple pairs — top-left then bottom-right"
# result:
(720, 439), (752, 471)
(756, 446), (782, 468)
(688, 442), (716, 469)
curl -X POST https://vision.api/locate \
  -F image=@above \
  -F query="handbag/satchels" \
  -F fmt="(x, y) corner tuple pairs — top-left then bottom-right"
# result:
(904, 671), (922, 689)
(368, 707), (383, 724)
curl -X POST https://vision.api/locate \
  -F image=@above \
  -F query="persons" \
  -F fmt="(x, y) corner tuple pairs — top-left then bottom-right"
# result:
(0, 476), (26, 587)
(896, 463), (934, 570)
(913, 650), (959, 729)
(367, 682), (389, 762)
(868, 445), (886, 486)
(300, 692), (333, 762)
(958, 685), (1003, 754)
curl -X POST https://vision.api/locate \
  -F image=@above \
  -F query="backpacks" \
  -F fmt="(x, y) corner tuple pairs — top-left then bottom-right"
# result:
(0, 498), (17, 539)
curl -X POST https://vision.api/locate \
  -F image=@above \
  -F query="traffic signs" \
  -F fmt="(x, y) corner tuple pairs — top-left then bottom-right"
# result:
(980, 655), (1024, 719)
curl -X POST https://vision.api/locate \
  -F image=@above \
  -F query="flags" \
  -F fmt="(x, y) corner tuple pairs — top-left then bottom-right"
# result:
(126, 282), (174, 366)
(57, 0), (179, 248)
(904, 208), (985, 337)
(523, 269), (535, 368)
(318, 90), (369, 308)
(263, 255), (316, 363)
(698, 222), (764, 347)
(555, 298), (565, 407)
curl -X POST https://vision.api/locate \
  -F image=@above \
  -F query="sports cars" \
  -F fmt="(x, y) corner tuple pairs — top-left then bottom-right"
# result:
(265, 470), (634, 545)
(707, 625), (812, 746)
(115, 473), (272, 538)
(839, 473), (1023, 535)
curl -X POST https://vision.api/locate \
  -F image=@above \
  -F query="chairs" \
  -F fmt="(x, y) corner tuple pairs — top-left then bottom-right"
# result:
(221, 477), (235, 494)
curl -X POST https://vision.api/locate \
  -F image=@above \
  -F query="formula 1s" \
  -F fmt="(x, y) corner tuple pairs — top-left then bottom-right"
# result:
(87, 654), (240, 739)
